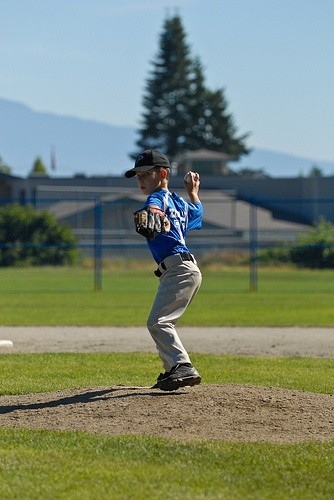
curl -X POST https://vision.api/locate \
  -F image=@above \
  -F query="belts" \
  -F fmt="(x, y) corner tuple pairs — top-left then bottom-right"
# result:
(154, 252), (193, 277)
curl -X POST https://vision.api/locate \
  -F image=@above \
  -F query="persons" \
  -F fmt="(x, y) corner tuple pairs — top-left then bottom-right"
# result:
(125, 148), (203, 391)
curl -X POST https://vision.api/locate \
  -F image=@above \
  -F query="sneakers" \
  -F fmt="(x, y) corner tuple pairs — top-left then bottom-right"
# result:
(159, 363), (202, 391)
(149, 372), (173, 389)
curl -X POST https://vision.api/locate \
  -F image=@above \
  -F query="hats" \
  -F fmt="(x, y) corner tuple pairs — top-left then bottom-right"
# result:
(125, 149), (171, 178)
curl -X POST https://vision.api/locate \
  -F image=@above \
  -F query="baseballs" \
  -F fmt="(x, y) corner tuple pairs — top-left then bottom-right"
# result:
(184, 172), (197, 182)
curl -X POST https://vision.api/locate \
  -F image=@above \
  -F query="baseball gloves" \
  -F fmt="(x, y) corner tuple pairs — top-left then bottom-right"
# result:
(133, 209), (171, 238)
